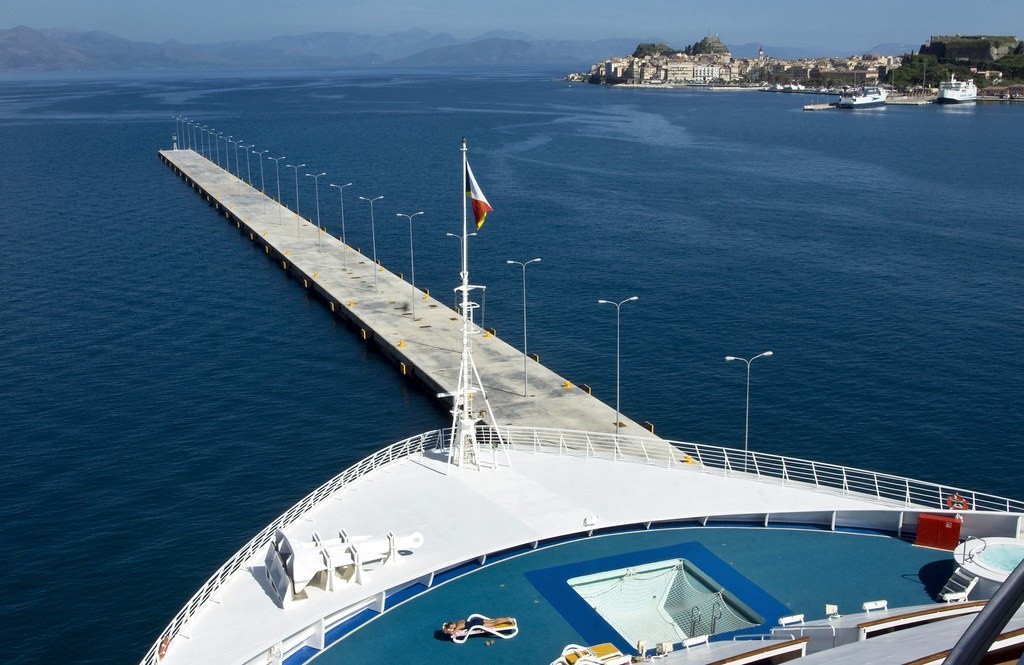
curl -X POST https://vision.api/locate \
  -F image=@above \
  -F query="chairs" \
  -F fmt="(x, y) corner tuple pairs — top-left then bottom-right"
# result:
(443, 613), (519, 644)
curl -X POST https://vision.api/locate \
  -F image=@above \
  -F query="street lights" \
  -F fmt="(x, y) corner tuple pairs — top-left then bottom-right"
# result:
(252, 150), (269, 215)
(195, 125), (207, 157)
(267, 156), (286, 225)
(396, 210), (425, 319)
(239, 145), (256, 199)
(286, 163), (306, 238)
(171, 115), (193, 151)
(330, 183), (353, 271)
(359, 195), (384, 292)
(446, 232), (478, 284)
(202, 128), (215, 161)
(210, 132), (223, 168)
(305, 173), (327, 253)
(228, 139), (244, 183)
(724, 350), (774, 475)
(507, 258), (542, 398)
(598, 295), (640, 448)
(219, 135), (234, 175)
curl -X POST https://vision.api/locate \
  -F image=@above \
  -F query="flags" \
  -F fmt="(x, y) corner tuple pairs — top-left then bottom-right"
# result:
(465, 159), (492, 232)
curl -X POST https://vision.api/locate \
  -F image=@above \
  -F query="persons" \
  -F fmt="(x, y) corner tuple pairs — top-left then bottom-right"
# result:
(443, 617), (513, 635)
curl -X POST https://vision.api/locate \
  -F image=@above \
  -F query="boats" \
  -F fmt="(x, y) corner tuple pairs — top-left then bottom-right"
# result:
(934, 72), (979, 103)
(834, 85), (889, 110)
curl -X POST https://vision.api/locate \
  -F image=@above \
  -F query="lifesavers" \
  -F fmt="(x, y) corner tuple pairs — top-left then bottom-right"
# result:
(947, 495), (967, 510)
(158, 635), (169, 657)
